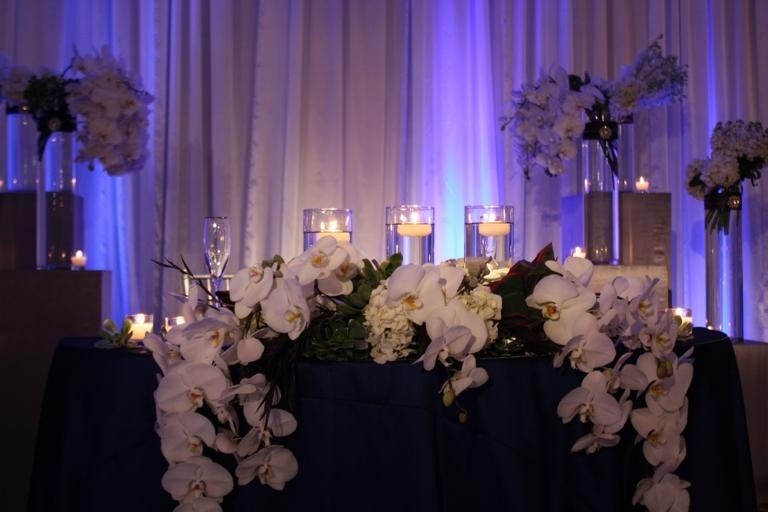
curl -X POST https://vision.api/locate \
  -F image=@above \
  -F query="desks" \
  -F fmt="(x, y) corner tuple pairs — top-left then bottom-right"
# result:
(51, 326), (736, 512)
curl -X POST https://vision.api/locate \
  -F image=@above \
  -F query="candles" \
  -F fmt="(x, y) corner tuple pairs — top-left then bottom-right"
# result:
(572, 244), (587, 260)
(315, 220), (352, 244)
(477, 211), (510, 237)
(71, 249), (87, 267)
(397, 208), (432, 238)
(130, 311), (153, 341)
(633, 174), (650, 192)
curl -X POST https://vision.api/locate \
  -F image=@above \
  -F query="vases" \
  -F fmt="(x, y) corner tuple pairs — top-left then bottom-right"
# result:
(704, 186), (745, 344)
(581, 121), (619, 266)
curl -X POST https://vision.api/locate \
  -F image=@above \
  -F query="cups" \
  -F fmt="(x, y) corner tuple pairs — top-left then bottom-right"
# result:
(570, 247), (588, 260)
(301, 205), (354, 252)
(462, 204), (518, 269)
(70, 248), (88, 271)
(635, 175), (653, 194)
(122, 313), (155, 346)
(664, 305), (694, 338)
(163, 316), (187, 335)
(383, 202), (435, 264)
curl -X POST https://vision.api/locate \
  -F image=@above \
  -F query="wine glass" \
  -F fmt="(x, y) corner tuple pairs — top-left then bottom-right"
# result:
(203, 215), (233, 308)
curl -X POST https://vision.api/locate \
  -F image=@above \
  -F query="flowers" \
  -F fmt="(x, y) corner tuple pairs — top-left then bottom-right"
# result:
(497, 31), (689, 182)
(685, 117), (768, 235)
(0, 42), (156, 178)
(141, 234), (696, 512)
